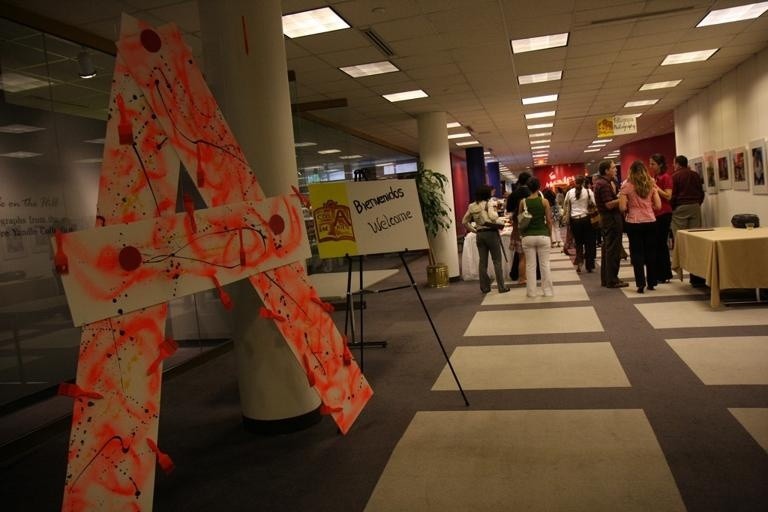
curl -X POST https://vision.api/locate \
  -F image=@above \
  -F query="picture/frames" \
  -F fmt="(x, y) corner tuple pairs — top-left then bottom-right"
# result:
(689, 138), (767, 196)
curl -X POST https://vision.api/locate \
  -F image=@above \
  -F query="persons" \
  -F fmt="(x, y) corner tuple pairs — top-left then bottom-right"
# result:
(459, 150), (704, 299)
(707, 147), (765, 189)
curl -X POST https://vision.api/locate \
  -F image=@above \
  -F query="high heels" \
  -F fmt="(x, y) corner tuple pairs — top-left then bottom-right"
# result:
(576, 264), (582, 272)
(637, 285), (643, 293)
(647, 282), (654, 290)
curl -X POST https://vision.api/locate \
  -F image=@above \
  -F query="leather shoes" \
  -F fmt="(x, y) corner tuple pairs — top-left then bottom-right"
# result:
(618, 279), (624, 283)
(563, 248), (569, 255)
(613, 280), (628, 287)
(504, 287), (510, 291)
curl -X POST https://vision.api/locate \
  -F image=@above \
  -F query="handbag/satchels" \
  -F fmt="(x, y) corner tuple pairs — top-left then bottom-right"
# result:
(480, 200), (505, 230)
(517, 198), (533, 229)
(587, 190), (600, 226)
(562, 192), (570, 225)
(619, 188), (628, 212)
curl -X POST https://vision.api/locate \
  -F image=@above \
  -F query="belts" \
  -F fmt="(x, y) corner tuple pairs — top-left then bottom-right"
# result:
(572, 214), (587, 219)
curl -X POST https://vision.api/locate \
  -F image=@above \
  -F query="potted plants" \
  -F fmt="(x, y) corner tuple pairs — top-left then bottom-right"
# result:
(410, 161), (456, 290)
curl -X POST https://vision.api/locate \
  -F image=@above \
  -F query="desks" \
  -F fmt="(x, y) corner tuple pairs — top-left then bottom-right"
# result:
(667, 223), (768, 310)
(463, 218), (517, 278)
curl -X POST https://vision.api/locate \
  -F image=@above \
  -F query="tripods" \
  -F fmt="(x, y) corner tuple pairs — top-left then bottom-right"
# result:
(343, 252), (470, 407)
